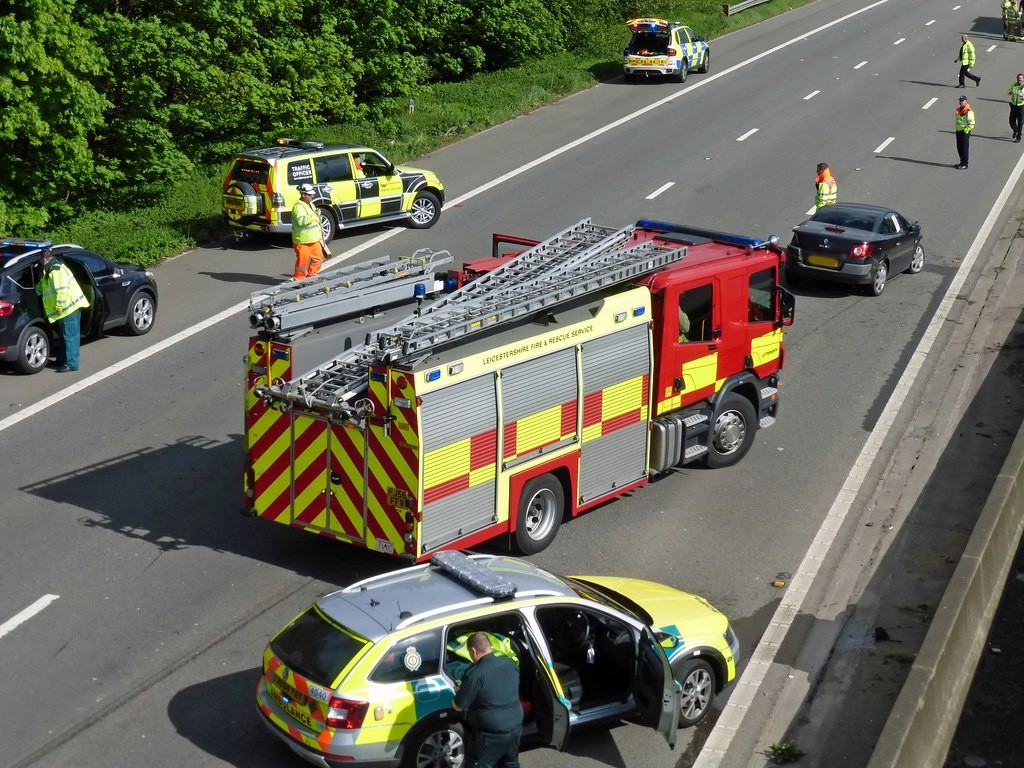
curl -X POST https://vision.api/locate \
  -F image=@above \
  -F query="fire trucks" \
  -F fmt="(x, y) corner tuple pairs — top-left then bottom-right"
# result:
(237, 217), (796, 566)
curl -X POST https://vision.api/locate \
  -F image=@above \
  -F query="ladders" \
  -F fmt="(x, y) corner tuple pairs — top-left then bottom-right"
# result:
(247, 248), (455, 342)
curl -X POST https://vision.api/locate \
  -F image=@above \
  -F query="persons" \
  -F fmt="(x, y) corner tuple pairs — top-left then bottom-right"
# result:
(34, 251), (90, 373)
(1000, 0), (1024, 42)
(679, 306), (691, 344)
(291, 184), (324, 281)
(1008, 74), (1024, 143)
(814, 163), (837, 211)
(353, 154), (366, 178)
(954, 96), (976, 169)
(452, 632), (523, 768)
(954, 34), (981, 88)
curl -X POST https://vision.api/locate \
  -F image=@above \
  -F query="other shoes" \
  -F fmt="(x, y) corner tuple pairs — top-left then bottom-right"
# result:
(1015, 137), (1021, 143)
(1009, 38), (1016, 42)
(956, 85), (965, 88)
(1019, 36), (1024, 41)
(954, 163), (968, 169)
(1005, 37), (1009, 40)
(1013, 130), (1017, 138)
(976, 77), (980, 86)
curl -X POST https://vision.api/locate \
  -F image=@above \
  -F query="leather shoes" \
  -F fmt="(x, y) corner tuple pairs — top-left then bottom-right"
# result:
(50, 361), (64, 368)
(57, 366), (78, 372)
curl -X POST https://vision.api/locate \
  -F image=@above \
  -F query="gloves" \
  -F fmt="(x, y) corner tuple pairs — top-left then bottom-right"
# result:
(315, 208), (321, 215)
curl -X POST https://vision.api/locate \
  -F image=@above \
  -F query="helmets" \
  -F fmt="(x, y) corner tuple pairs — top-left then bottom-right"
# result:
(300, 184), (316, 194)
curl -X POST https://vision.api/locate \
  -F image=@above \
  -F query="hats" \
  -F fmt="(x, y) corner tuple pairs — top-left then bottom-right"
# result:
(958, 96), (967, 100)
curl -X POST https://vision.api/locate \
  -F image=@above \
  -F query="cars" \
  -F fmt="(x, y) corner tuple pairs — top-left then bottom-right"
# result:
(786, 202), (923, 296)
(248, 545), (744, 768)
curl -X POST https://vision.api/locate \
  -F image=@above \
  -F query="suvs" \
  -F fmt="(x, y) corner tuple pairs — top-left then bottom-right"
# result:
(221, 138), (446, 246)
(0, 236), (159, 375)
(622, 18), (711, 83)
